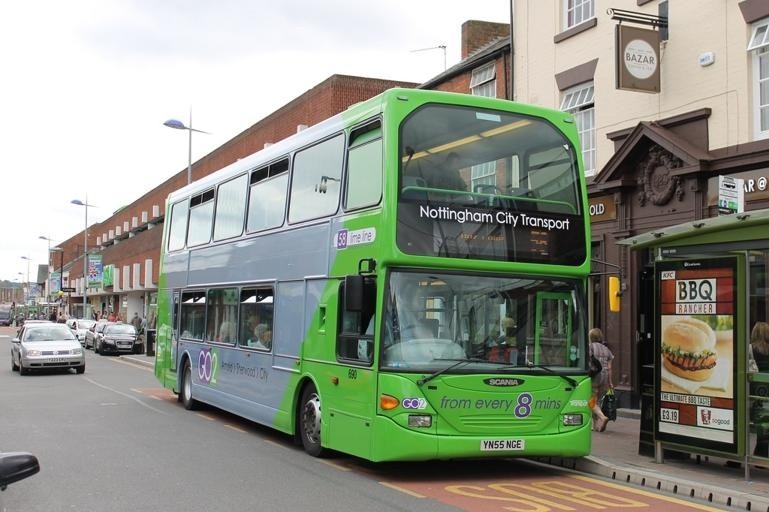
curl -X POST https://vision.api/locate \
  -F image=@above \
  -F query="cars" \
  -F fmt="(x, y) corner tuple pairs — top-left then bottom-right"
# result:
(11, 318), (144, 375)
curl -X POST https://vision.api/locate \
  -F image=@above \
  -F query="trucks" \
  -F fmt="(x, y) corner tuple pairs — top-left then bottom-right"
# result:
(0, 301), (16, 326)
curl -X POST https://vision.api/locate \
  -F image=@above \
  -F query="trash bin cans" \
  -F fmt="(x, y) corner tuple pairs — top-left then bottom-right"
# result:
(147, 329), (156, 356)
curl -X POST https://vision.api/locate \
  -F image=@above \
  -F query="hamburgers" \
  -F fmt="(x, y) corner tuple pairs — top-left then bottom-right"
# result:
(661, 316), (717, 381)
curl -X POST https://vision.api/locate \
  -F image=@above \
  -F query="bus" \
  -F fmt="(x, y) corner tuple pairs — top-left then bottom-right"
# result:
(154, 87), (594, 465)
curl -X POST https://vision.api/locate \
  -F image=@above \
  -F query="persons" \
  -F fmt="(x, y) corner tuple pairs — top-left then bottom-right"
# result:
(430, 151), (466, 199)
(357, 287), (439, 359)
(92, 309), (148, 331)
(213, 305), (271, 349)
(747, 321), (769, 373)
(31, 310), (56, 321)
(590, 328), (616, 434)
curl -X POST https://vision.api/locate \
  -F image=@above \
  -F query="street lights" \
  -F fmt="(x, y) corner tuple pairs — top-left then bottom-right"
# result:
(38, 236), (55, 320)
(71, 196), (97, 318)
(19, 256), (31, 291)
(163, 113), (208, 185)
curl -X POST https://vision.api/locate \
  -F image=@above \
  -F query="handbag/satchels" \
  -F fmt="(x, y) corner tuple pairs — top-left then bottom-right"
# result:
(589, 343), (602, 377)
(601, 388), (616, 423)
